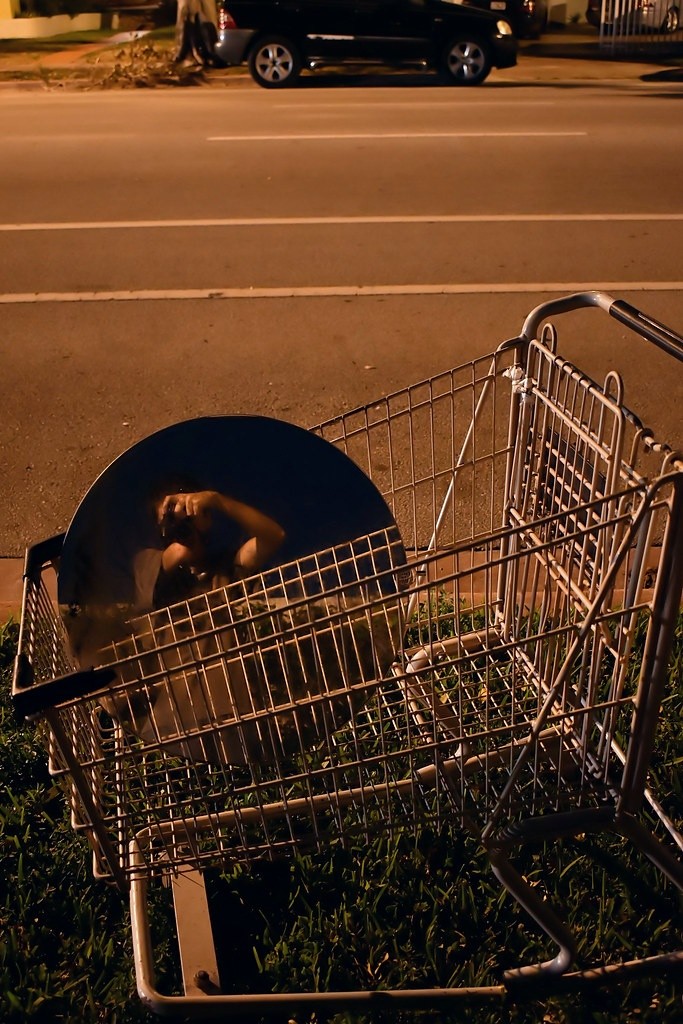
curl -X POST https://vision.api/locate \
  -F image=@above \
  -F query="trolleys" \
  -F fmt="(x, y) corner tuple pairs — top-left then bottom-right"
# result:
(9, 291), (682, 1022)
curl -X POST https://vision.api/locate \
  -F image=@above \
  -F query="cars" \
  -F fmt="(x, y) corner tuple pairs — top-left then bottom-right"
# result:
(461, 0), (547, 39)
(586, 0), (683, 35)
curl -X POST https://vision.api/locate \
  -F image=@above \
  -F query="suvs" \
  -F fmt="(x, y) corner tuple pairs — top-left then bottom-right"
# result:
(188, 0), (518, 90)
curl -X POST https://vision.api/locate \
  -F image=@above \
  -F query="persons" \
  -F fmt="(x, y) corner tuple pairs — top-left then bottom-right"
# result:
(130, 484), (286, 761)
